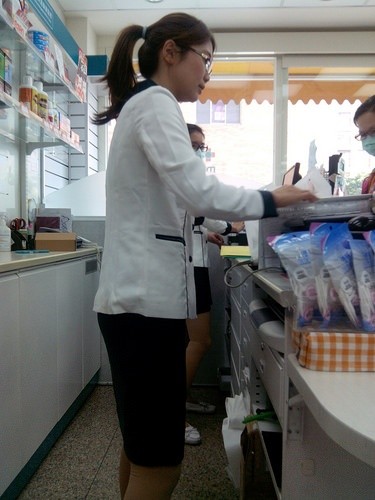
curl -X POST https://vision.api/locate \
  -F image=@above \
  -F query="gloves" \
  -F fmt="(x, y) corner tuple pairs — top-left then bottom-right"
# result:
(372, 190), (375, 214)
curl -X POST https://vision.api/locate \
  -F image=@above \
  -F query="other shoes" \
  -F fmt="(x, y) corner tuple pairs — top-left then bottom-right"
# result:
(185, 423), (199, 444)
(185, 401), (215, 415)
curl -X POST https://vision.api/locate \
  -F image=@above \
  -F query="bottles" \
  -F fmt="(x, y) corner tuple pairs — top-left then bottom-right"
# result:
(20, 77), (60, 129)
(0, 219), (11, 253)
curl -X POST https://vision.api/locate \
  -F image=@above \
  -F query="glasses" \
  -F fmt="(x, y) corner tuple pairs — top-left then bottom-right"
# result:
(355, 129), (375, 141)
(176, 43), (212, 75)
(192, 143), (208, 151)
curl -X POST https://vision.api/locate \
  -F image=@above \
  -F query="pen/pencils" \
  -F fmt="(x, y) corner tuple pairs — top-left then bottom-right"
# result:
(16, 250), (49, 253)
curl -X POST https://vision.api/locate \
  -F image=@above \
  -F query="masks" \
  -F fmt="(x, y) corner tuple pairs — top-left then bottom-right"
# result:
(362, 135), (375, 156)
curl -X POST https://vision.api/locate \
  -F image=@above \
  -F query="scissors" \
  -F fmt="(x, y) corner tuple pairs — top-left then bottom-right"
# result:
(10, 218), (25, 230)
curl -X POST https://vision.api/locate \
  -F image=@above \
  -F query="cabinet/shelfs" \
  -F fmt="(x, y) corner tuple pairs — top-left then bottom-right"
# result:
(0, 255), (102, 500)
(0, 5), (88, 156)
(228, 268), (375, 500)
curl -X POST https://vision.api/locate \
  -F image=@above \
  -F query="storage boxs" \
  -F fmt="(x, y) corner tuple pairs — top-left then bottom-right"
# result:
(36, 233), (77, 251)
(291, 318), (375, 373)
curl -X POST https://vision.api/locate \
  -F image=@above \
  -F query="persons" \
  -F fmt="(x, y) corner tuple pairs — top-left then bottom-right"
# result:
(93, 12), (320, 500)
(178, 124), (247, 446)
(353, 94), (375, 195)
